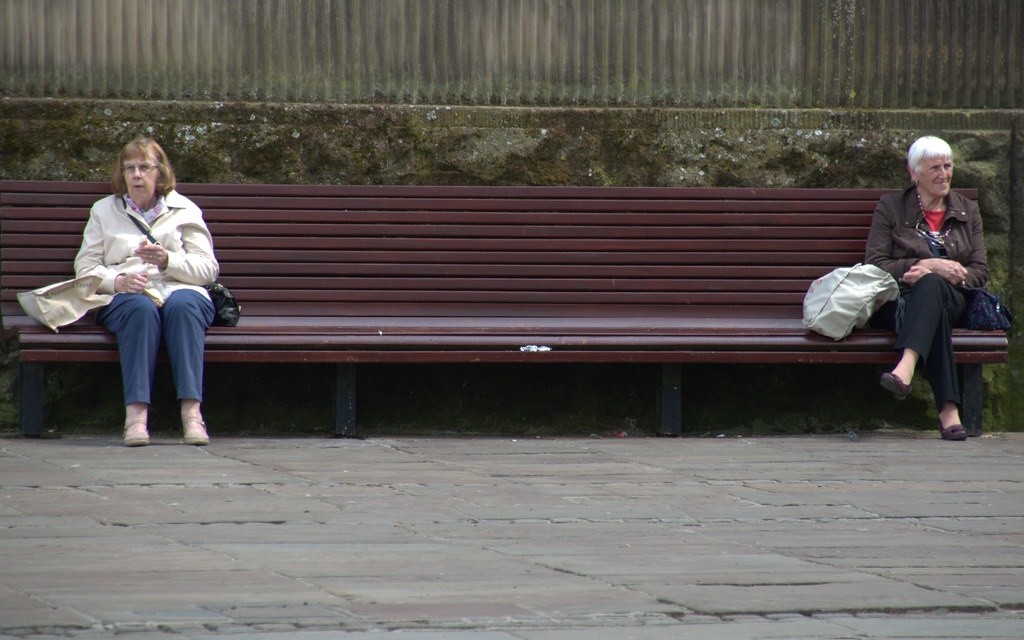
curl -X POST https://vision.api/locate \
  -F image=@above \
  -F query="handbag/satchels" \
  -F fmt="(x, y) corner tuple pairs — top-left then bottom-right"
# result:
(17, 274), (112, 334)
(960, 285), (1013, 331)
(801, 260), (900, 342)
(203, 284), (241, 328)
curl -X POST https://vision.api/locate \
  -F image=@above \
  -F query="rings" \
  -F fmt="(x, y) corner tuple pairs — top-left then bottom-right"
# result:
(150, 255), (152, 260)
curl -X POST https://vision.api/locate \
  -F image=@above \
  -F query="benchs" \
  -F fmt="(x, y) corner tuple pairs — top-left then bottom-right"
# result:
(0, 180), (1008, 440)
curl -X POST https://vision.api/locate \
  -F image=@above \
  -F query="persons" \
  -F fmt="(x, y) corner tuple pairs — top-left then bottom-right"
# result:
(863, 137), (986, 440)
(75, 138), (218, 447)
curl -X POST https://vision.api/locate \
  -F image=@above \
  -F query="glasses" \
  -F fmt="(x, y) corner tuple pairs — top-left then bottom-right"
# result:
(121, 162), (159, 172)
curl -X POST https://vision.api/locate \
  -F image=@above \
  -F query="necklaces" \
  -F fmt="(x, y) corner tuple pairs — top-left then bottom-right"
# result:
(916, 190), (954, 238)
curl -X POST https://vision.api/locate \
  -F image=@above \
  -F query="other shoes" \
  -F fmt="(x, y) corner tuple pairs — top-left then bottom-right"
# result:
(878, 372), (912, 401)
(122, 420), (149, 447)
(182, 417), (209, 444)
(939, 417), (968, 439)
